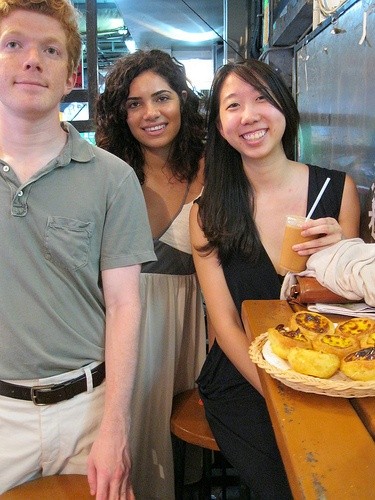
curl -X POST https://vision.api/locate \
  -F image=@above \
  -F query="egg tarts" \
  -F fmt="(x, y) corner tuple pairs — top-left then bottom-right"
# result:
(267, 311), (375, 382)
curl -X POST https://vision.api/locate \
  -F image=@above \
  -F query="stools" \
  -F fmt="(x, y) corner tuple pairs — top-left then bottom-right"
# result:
(0, 474), (99, 500)
(170, 387), (251, 500)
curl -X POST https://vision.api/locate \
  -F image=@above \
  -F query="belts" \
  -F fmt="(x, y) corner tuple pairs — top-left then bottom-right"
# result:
(0, 361), (108, 406)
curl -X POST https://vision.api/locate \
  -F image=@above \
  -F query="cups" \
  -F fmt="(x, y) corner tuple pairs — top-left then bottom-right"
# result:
(278, 214), (321, 274)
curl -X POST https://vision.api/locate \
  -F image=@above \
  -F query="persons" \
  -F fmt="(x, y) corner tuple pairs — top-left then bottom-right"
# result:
(188, 59), (361, 500)
(0, 0), (159, 500)
(93, 48), (209, 500)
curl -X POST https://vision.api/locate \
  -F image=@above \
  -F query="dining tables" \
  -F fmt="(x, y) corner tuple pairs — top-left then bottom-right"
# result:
(242, 300), (375, 500)
